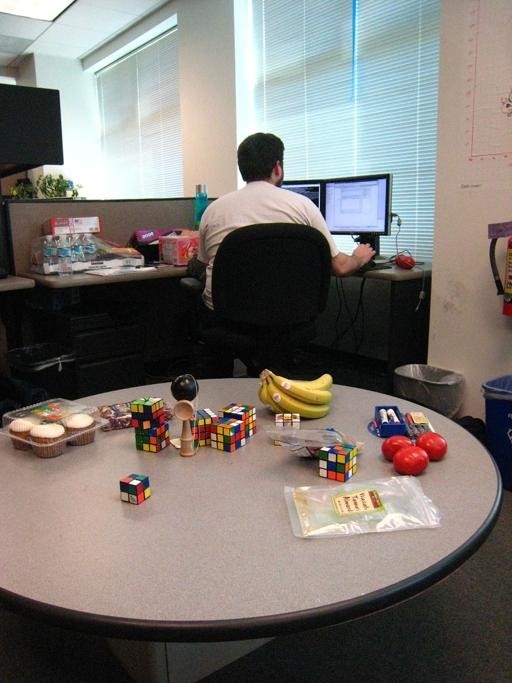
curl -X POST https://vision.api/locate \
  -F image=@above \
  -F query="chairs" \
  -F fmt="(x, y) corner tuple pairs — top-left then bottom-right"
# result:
(192, 225), (333, 369)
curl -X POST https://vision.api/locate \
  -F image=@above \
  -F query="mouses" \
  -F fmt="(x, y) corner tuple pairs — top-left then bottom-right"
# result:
(366, 260), (377, 268)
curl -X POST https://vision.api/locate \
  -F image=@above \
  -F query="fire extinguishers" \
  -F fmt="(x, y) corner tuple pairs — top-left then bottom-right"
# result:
(488, 237), (512, 316)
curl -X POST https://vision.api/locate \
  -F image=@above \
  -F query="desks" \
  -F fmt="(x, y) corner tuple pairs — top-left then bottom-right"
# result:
(15, 256), (431, 401)
(1, 374), (502, 683)
(0, 273), (36, 349)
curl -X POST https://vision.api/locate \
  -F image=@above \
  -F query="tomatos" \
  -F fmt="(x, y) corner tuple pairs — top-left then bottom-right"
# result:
(416, 432), (448, 461)
(382, 435), (414, 462)
(394, 447), (429, 475)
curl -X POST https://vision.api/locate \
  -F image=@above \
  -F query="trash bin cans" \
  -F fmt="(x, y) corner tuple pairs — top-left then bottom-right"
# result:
(394, 364), (464, 418)
(482, 374), (512, 493)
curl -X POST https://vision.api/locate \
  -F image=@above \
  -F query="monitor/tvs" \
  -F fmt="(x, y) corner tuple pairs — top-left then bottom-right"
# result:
(322, 173), (394, 258)
(279, 178), (324, 216)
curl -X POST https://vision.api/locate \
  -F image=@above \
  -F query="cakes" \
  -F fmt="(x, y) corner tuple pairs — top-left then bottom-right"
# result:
(29, 423), (67, 458)
(63, 414), (96, 446)
(10, 417), (41, 451)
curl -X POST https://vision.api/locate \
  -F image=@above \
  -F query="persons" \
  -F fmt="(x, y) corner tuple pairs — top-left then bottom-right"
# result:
(196, 131), (376, 314)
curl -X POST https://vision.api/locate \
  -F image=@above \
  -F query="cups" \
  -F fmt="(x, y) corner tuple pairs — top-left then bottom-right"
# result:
(58, 257), (73, 278)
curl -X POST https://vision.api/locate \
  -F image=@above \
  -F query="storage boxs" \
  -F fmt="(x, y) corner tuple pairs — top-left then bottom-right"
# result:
(159, 236), (201, 265)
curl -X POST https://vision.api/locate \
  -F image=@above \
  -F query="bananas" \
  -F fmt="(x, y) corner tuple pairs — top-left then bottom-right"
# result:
(259, 368), (333, 419)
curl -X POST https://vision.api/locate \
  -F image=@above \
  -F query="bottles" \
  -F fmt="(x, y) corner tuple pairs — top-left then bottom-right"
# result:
(41, 235), (57, 264)
(57, 235), (72, 264)
(71, 234), (83, 262)
(83, 233), (96, 262)
(194, 184), (208, 231)
